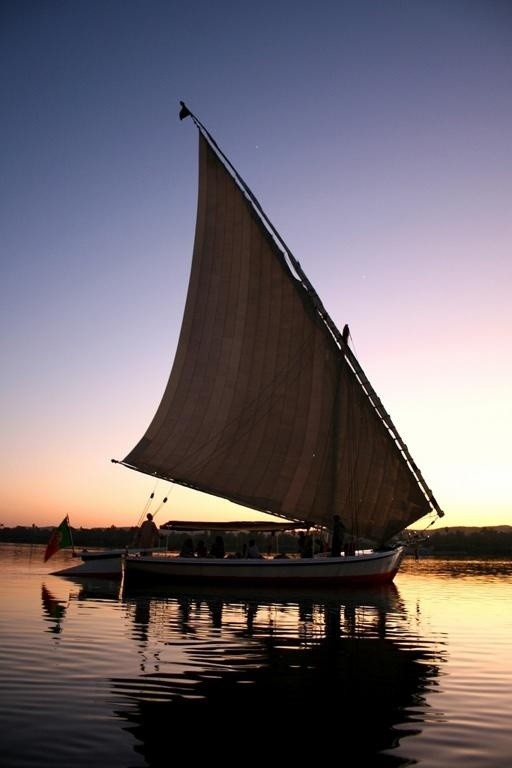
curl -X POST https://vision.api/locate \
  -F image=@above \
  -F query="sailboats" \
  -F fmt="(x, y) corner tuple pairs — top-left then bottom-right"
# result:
(51, 101), (442, 585)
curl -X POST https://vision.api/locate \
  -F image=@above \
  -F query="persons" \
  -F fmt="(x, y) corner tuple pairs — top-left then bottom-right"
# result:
(191, 540), (208, 558)
(139, 513), (158, 557)
(298, 531), (313, 559)
(248, 540), (258, 558)
(180, 540), (194, 558)
(210, 537), (225, 558)
(241, 544), (248, 558)
(332, 514), (345, 557)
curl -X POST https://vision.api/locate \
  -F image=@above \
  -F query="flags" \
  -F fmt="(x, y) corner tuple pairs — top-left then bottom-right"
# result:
(45, 516), (72, 563)
